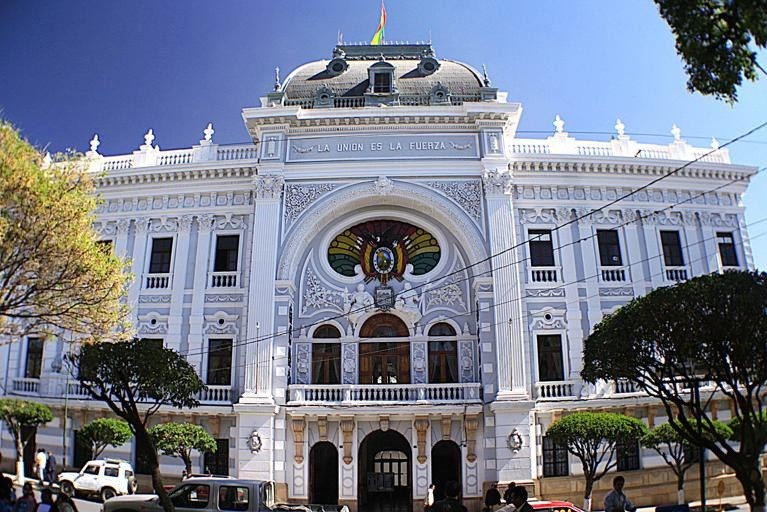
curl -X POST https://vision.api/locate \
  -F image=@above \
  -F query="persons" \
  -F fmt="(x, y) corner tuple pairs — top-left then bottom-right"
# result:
(0, 448), (78, 512)
(424, 482), (533, 512)
(397, 283), (422, 327)
(604, 476), (636, 512)
(348, 283), (374, 329)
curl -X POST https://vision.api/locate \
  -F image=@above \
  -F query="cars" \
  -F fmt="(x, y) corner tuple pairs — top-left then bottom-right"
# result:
(153, 473), (246, 506)
(494, 500), (588, 512)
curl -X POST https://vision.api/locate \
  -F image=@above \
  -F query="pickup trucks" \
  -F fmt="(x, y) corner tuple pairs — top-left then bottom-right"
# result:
(98, 477), (349, 512)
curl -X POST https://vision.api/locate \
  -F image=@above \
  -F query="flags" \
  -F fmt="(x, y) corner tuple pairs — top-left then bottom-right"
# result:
(371, 2), (386, 46)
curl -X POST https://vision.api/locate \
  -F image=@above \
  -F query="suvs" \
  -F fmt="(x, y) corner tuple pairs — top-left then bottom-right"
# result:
(57, 458), (137, 503)
